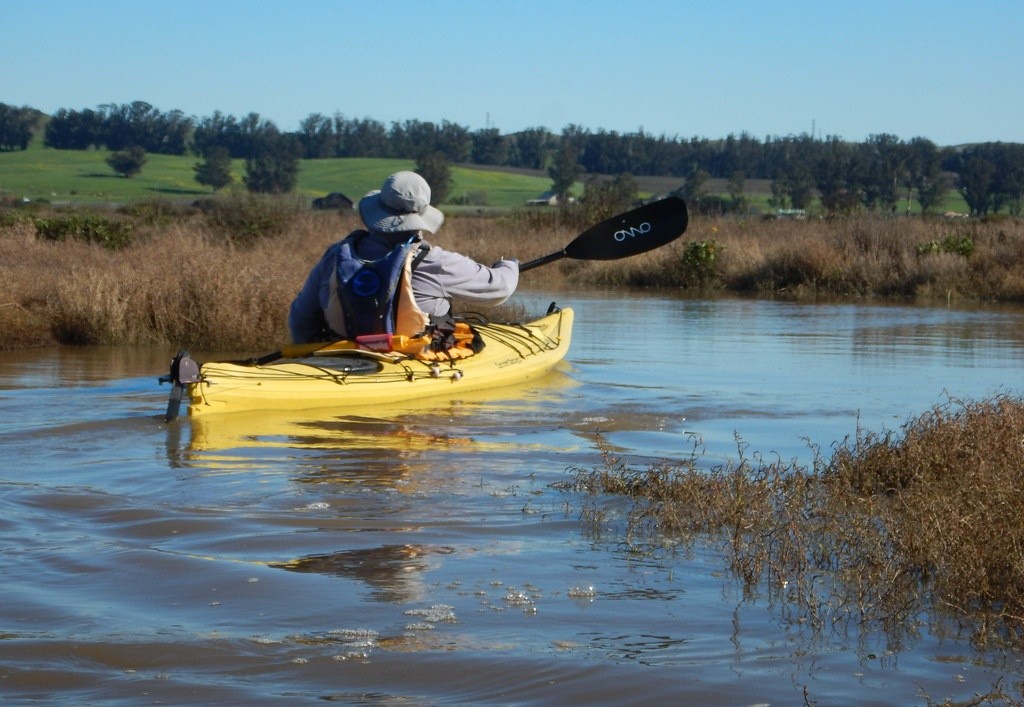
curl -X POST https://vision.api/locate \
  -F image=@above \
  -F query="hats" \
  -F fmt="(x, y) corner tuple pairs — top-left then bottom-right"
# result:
(358, 170), (445, 235)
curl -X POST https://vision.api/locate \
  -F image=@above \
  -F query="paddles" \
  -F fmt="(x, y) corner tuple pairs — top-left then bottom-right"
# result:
(158, 194), (688, 384)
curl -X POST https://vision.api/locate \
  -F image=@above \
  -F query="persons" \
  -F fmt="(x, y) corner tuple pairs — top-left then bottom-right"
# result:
(288, 170), (520, 346)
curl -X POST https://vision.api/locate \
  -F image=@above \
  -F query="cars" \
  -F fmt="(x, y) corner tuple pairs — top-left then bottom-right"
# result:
(525, 191), (575, 206)
(313, 192), (353, 208)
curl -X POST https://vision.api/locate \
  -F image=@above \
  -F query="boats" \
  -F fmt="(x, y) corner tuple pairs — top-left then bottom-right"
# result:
(161, 305), (574, 420)
